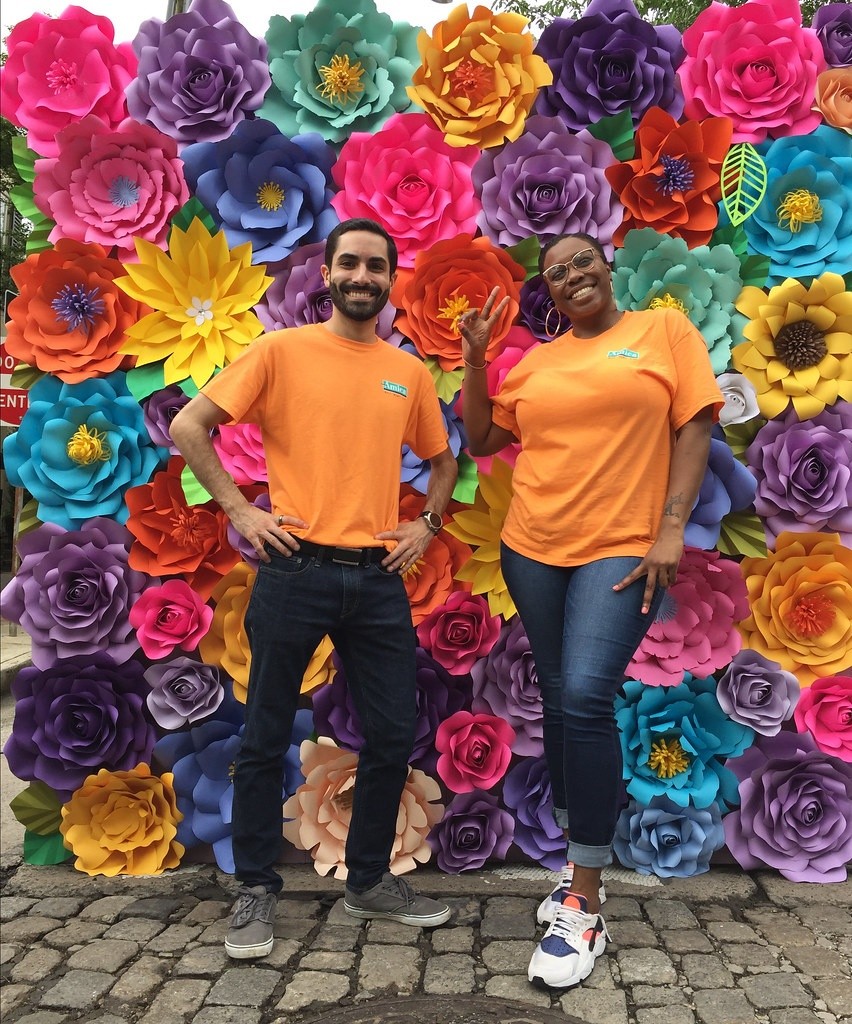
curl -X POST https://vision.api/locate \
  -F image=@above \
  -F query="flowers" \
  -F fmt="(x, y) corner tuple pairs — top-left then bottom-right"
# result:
(0, 0), (852, 885)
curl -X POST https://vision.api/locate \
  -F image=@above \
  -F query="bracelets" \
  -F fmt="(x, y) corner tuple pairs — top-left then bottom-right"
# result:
(462, 357), (487, 370)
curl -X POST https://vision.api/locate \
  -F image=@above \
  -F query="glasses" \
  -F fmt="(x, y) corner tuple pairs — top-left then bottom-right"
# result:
(542, 248), (605, 286)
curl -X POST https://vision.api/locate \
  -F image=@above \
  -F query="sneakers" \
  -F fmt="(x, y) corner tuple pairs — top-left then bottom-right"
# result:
(528, 891), (613, 993)
(536, 866), (607, 928)
(224, 885), (277, 959)
(344, 871), (451, 927)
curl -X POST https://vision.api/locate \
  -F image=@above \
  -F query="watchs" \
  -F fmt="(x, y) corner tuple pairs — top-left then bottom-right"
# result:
(417, 511), (443, 536)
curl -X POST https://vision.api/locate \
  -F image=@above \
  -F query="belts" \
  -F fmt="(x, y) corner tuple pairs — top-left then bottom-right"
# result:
(267, 532), (389, 566)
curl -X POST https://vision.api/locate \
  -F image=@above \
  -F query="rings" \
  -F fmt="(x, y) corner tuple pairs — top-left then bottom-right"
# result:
(279, 515), (284, 525)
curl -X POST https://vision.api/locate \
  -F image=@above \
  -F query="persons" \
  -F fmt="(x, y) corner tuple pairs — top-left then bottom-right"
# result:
(169, 218), (459, 958)
(456, 232), (726, 988)
